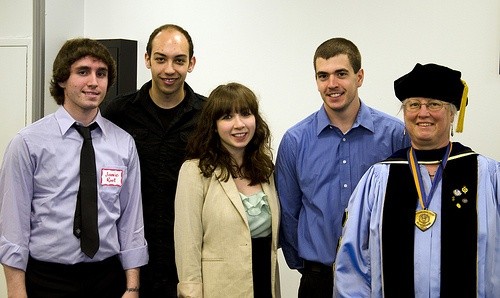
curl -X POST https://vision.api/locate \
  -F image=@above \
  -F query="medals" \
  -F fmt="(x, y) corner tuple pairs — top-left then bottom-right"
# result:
(414, 210), (437, 232)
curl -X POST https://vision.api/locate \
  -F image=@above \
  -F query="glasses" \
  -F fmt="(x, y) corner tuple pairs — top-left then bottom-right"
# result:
(404, 99), (450, 113)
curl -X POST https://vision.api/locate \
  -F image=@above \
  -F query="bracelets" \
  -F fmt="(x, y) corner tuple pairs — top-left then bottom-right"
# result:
(127, 288), (139, 292)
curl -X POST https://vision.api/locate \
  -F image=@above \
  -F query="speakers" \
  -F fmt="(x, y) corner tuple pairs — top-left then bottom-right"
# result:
(93, 39), (137, 112)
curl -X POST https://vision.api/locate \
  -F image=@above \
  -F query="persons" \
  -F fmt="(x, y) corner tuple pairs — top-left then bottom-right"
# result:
(174, 82), (281, 298)
(107, 24), (209, 298)
(333, 62), (500, 297)
(0, 38), (149, 298)
(275, 37), (412, 298)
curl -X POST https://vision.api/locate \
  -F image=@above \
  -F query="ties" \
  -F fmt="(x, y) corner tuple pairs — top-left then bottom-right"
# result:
(70, 123), (100, 260)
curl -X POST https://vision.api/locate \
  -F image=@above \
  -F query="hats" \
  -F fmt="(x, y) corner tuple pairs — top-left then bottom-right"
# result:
(394, 62), (469, 134)
